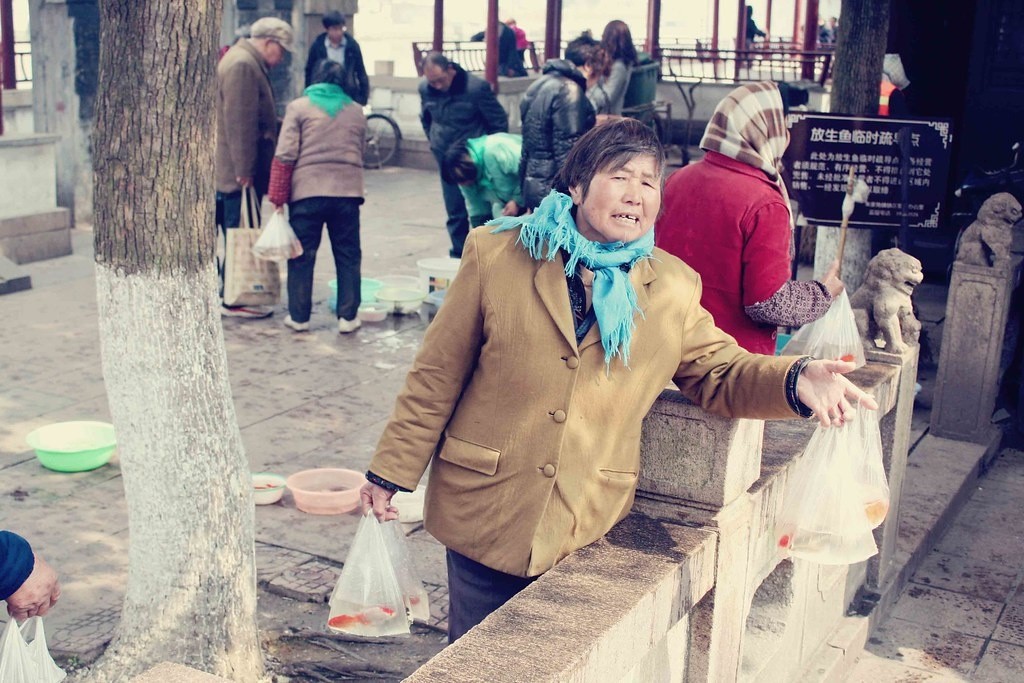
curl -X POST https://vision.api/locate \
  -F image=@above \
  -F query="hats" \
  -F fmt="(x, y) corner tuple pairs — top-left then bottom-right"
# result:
(250, 16), (299, 53)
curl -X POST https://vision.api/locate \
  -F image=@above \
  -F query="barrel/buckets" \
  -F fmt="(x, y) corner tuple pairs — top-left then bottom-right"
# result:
(416, 258), (461, 304)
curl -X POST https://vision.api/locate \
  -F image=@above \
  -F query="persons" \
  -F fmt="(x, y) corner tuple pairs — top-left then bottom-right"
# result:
(432, 130), (536, 229)
(743, 5), (770, 69)
(360, 118), (879, 647)
(470, 17), (530, 78)
(0, 531), (63, 620)
(517, 18), (640, 215)
(217, 16), (299, 319)
(799, 15), (839, 78)
(268, 60), (368, 335)
(654, 78), (845, 356)
(218, 23), (251, 61)
(304, 9), (370, 107)
(418, 50), (510, 259)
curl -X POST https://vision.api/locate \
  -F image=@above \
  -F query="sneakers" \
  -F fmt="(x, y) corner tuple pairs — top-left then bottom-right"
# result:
(220, 303), (274, 317)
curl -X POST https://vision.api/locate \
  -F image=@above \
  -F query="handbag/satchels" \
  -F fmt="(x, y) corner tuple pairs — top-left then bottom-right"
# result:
(223, 183), (282, 308)
(252, 210), (303, 261)
(777, 284), (867, 368)
(0, 615), (66, 683)
(767, 395), (890, 566)
(326, 508), (430, 638)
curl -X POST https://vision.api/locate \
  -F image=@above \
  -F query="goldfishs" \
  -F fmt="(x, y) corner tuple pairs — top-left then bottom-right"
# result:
(779, 531), (797, 548)
(327, 596), (419, 630)
(834, 352), (854, 363)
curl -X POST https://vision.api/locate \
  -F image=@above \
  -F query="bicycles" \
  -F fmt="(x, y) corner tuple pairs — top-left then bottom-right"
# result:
(359, 105), (403, 170)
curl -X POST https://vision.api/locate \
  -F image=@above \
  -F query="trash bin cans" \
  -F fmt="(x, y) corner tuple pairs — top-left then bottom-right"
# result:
(624, 53), (662, 129)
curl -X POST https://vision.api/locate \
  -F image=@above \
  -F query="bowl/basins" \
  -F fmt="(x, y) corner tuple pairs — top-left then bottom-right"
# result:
(390, 484), (426, 522)
(430, 291), (447, 308)
(251, 473), (286, 505)
(357, 303), (392, 322)
(329, 297), (337, 311)
(375, 288), (427, 314)
(27, 420), (116, 472)
(286, 468), (369, 515)
(328, 279), (382, 302)
(379, 276), (420, 290)
(775, 333), (792, 357)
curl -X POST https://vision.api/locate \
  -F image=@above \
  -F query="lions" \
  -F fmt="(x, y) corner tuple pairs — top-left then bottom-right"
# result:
(957, 192), (1022, 263)
(849, 247), (924, 354)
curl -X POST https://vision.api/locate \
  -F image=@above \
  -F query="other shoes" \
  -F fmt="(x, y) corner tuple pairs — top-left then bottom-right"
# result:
(339, 316), (362, 332)
(285, 314), (309, 330)
